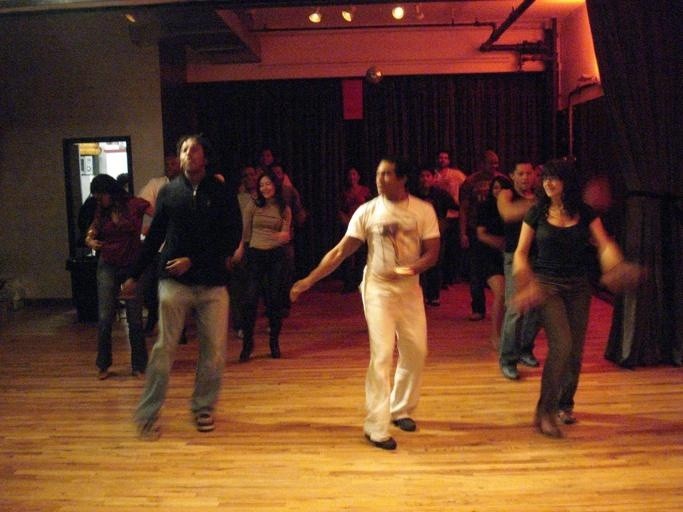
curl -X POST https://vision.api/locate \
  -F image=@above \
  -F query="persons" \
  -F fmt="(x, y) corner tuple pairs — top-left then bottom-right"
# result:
(496, 151), (557, 380)
(336, 167), (366, 289)
(422, 152), (513, 321)
(507, 160), (620, 438)
(69, 149), (187, 344)
(115, 136), (243, 441)
(209, 149), (306, 362)
(289, 154), (441, 450)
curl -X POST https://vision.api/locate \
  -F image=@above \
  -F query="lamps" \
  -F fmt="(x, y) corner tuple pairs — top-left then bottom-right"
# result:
(126, 6), (143, 23)
(414, 5), (424, 20)
(342, 5), (357, 22)
(309, 6), (322, 23)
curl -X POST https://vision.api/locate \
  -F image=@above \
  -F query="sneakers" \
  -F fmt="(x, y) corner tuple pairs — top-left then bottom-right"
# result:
(520, 354), (538, 367)
(501, 364), (519, 379)
(559, 411), (575, 424)
(533, 422), (562, 439)
(428, 283), (484, 321)
(364, 433), (396, 450)
(193, 411), (214, 431)
(394, 417), (416, 432)
(95, 302), (291, 381)
